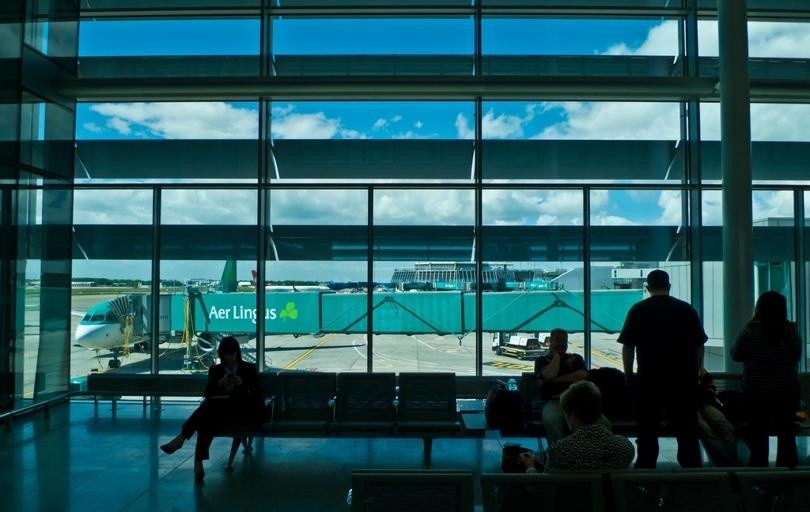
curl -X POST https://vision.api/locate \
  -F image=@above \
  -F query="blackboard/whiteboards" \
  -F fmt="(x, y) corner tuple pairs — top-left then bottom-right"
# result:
(489, 327), (554, 360)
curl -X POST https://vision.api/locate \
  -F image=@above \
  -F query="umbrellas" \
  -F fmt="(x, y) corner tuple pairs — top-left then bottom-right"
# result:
(159, 442), (183, 454)
(194, 466), (205, 481)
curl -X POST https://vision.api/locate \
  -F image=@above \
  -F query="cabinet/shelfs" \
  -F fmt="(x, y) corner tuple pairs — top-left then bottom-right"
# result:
(507, 379), (517, 390)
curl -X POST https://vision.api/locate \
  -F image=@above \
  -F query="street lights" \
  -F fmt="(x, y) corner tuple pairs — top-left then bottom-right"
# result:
(483, 382), (524, 437)
(502, 445), (545, 472)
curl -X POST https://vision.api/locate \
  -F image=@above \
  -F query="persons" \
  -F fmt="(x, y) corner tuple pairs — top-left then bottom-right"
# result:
(533, 328), (613, 448)
(699, 364), (752, 467)
(518, 380), (636, 474)
(616, 270), (710, 470)
(731, 291), (802, 467)
(159, 335), (257, 482)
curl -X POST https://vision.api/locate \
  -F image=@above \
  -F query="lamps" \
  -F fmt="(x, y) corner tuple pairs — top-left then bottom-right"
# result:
(520, 371), (810, 452)
(226, 371), (466, 457)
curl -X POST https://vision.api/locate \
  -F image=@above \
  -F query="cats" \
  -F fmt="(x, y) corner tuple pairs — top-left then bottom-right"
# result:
(248, 268), (332, 295)
(71, 259), (260, 372)
